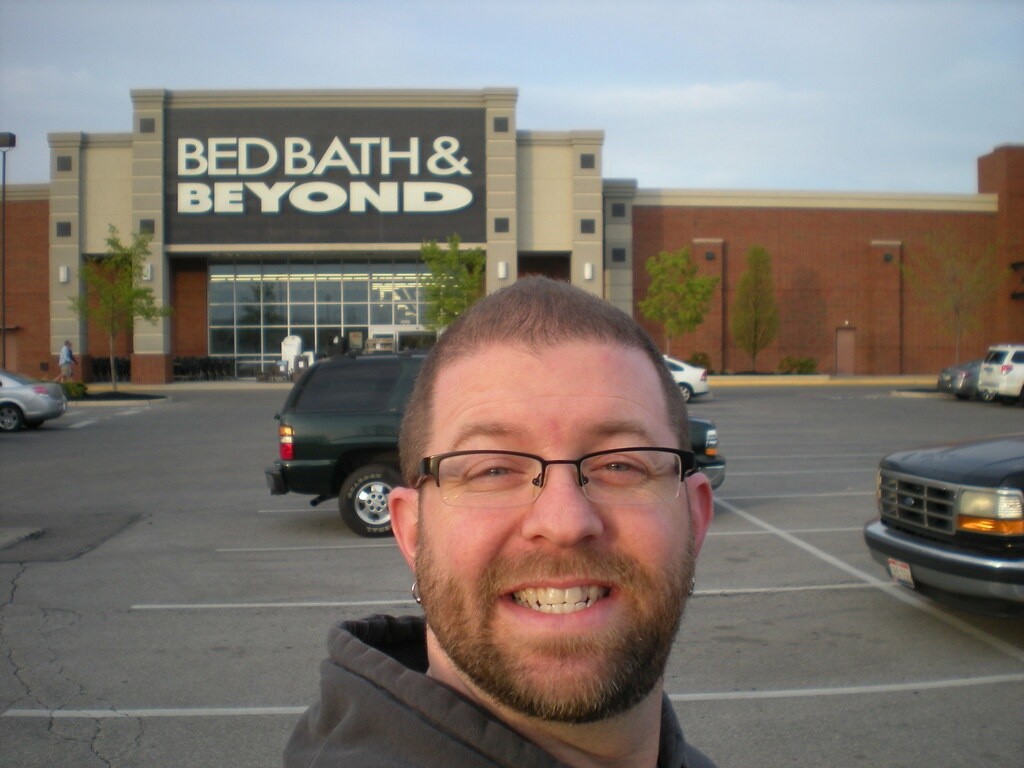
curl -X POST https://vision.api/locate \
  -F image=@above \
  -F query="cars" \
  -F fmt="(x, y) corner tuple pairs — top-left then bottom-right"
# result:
(0, 366), (69, 433)
(939, 357), (981, 401)
(864, 432), (1024, 609)
(665, 356), (712, 399)
(980, 342), (1024, 404)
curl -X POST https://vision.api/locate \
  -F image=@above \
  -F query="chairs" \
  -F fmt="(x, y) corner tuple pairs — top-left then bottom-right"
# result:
(270, 360), (288, 382)
(91, 355), (240, 381)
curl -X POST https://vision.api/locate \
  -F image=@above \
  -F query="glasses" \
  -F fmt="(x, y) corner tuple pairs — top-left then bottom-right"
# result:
(412, 446), (700, 509)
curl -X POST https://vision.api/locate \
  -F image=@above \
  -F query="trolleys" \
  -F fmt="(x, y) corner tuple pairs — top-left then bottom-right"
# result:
(89, 356), (240, 381)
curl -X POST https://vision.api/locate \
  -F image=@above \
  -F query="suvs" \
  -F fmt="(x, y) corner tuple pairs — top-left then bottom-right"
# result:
(264, 352), (728, 539)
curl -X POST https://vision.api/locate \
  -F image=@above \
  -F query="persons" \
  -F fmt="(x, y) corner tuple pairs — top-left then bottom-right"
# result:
(54, 340), (79, 383)
(281, 274), (723, 768)
